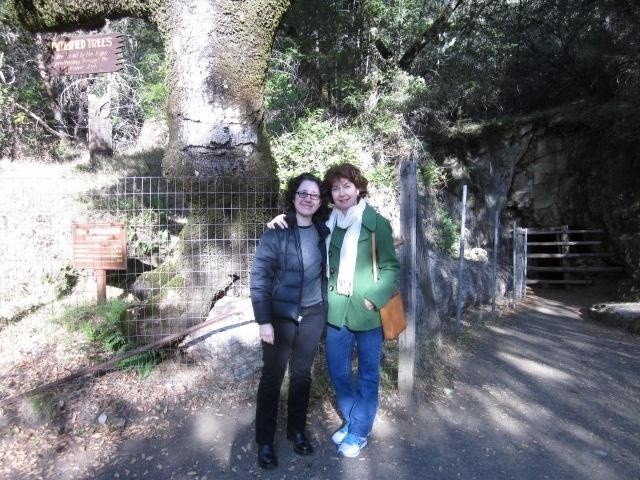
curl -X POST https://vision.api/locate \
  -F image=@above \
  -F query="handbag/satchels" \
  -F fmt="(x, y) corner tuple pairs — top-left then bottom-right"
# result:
(380, 292), (407, 340)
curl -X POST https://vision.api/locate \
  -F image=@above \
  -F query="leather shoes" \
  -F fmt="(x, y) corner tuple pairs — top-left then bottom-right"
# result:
(286, 429), (312, 456)
(258, 443), (278, 469)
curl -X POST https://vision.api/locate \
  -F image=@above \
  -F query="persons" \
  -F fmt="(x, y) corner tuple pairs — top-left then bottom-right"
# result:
(263, 162), (401, 458)
(247, 172), (327, 471)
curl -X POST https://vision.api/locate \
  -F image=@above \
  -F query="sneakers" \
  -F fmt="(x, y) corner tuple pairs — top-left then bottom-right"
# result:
(331, 426), (367, 457)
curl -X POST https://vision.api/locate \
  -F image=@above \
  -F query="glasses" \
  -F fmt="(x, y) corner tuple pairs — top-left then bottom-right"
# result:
(295, 191), (320, 200)
